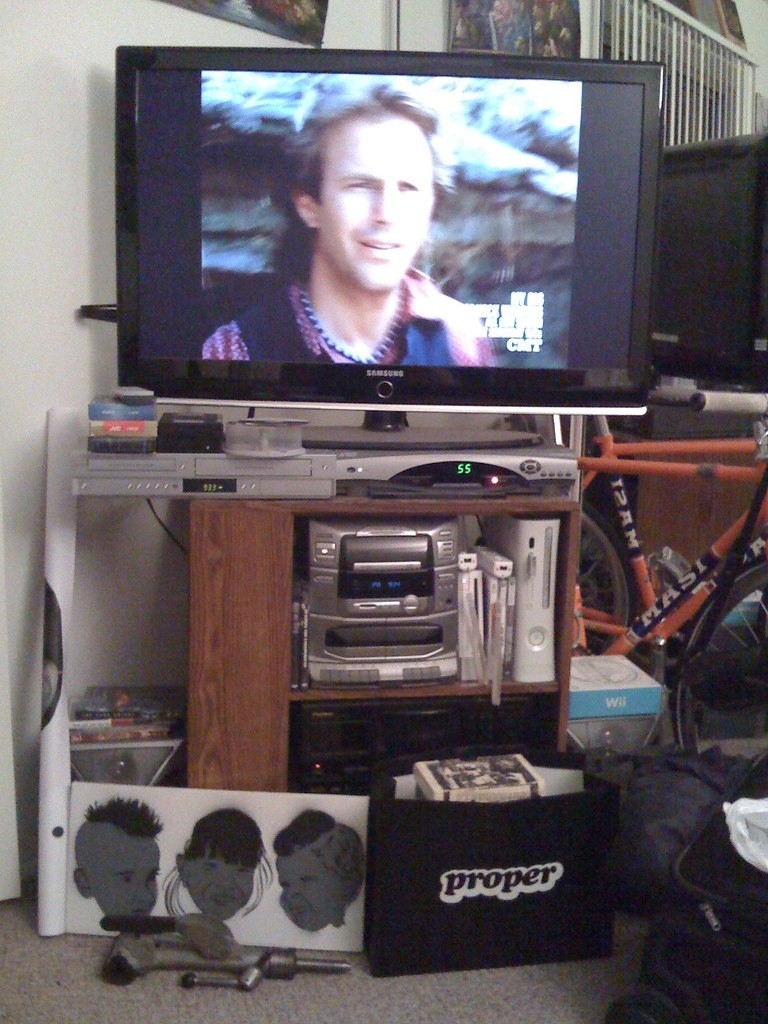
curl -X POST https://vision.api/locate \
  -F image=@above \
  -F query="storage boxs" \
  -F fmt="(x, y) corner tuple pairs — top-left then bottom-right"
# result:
(567, 654), (664, 721)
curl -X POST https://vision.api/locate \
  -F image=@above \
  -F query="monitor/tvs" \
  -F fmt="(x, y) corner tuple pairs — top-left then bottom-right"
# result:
(112, 45), (664, 446)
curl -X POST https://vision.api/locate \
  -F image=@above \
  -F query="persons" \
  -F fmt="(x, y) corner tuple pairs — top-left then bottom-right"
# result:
(200, 82), (498, 366)
(454, 0), (578, 60)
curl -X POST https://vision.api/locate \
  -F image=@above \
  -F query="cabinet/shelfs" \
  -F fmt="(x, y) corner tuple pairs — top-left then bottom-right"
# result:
(188, 495), (582, 791)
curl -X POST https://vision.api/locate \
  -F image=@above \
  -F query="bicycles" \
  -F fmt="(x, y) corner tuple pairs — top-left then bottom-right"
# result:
(569, 392), (767, 797)
